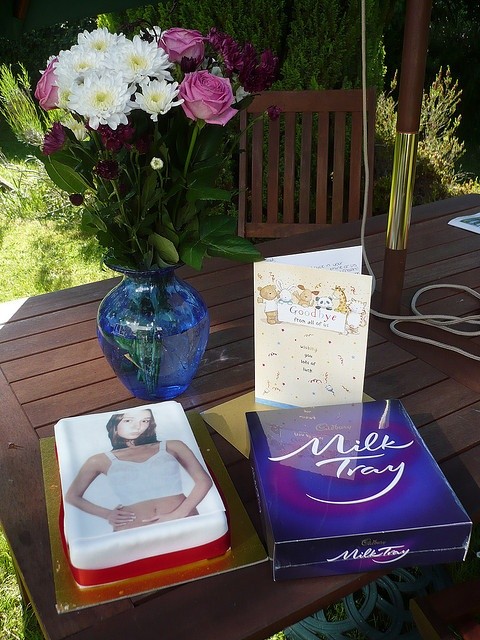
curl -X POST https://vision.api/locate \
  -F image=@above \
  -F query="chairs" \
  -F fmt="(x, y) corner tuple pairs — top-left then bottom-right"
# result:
(238, 91), (375, 244)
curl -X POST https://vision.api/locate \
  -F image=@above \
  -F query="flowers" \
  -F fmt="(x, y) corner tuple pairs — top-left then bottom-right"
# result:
(34, 28), (284, 382)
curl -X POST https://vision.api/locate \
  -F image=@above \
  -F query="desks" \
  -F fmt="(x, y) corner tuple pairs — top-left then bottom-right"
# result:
(1, 193), (480, 639)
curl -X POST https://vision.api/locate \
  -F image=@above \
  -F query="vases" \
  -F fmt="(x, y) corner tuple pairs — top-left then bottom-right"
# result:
(96, 251), (209, 401)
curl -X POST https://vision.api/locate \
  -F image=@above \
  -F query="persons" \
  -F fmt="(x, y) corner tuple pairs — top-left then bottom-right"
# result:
(65, 409), (214, 532)
